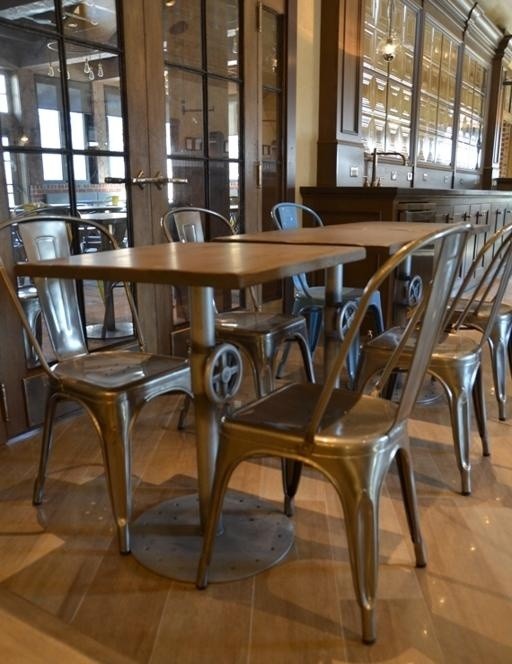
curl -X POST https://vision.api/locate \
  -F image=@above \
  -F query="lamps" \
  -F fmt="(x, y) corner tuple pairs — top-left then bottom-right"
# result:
(46, 29), (106, 84)
(381, 0), (396, 61)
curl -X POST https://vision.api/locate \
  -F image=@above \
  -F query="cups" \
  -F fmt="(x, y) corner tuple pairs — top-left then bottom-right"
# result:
(112, 196), (119, 207)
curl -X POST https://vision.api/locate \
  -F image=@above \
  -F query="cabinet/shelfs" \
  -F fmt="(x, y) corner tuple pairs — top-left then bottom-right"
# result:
(299, 185), (512, 333)
